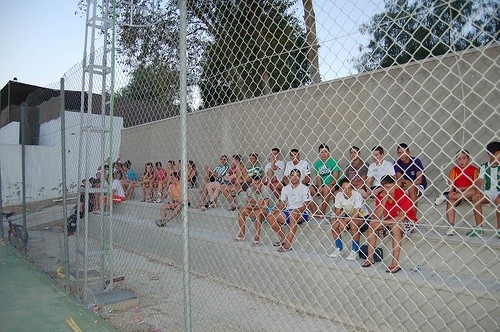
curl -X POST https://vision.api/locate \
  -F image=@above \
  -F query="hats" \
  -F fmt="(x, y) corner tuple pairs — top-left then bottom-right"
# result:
(80, 170), (108, 187)
(104, 163), (109, 167)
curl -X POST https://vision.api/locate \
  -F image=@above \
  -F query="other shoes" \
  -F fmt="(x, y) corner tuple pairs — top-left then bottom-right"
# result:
(156, 196), (162, 203)
(212, 202), (218, 208)
(194, 204), (200, 208)
(188, 202), (191, 207)
(103, 211), (111, 215)
(228, 203), (239, 211)
(92, 209), (100, 214)
(201, 205), (209, 211)
(236, 236), (245, 240)
(253, 240), (260, 244)
(148, 197), (153, 203)
(142, 197), (147, 202)
(155, 218), (166, 227)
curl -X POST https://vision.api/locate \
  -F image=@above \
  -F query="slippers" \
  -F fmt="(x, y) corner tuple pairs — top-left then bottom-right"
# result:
(278, 245), (292, 252)
(273, 240), (286, 246)
(386, 265), (401, 273)
(361, 259), (375, 267)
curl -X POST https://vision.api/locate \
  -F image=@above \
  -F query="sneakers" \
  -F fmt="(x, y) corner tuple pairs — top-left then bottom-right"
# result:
(466, 227), (484, 237)
(496, 232), (500, 239)
(346, 250), (359, 260)
(434, 191), (446, 206)
(330, 249), (343, 257)
(445, 226), (454, 235)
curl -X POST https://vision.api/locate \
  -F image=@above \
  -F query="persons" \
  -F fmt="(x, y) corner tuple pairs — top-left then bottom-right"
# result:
(361, 174), (418, 274)
(67, 140), (500, 273)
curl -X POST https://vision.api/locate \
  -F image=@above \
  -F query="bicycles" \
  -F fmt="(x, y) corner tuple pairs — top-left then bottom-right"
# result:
(2, 212), (28, 248)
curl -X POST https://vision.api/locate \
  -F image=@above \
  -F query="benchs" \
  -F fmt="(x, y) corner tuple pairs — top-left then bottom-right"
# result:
(72, 179), (500, 332)
